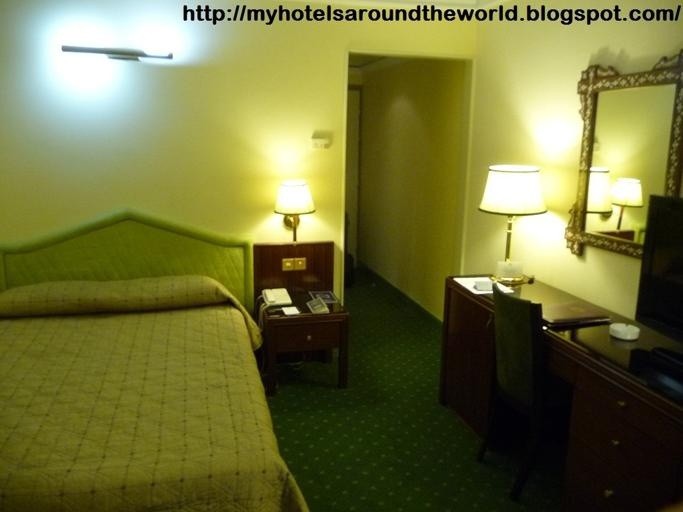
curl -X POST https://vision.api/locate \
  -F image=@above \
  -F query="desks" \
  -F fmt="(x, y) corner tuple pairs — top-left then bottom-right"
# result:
(439, 274), (683, 512)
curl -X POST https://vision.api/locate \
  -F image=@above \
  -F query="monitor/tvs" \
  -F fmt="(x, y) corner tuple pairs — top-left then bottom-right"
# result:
(629, 194), (683, 405)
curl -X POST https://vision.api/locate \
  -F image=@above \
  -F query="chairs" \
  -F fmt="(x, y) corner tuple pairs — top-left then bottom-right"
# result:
(478, 285), (578, 502)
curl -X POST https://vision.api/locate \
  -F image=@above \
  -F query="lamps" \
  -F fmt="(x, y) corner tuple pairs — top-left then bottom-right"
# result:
(612, 176), (644, 236)
(586, 165), (614, 215)
(478, 165), (547, 287)
(273, 180), (316, 241)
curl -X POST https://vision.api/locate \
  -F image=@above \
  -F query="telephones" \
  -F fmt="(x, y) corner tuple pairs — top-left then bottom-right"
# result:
(262, 287), (292, 307)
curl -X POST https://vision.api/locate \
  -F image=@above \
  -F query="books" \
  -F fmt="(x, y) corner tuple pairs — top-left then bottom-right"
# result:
(308, 290), (340, 304)
(306, 297), (330, 315)
(532, 298), (611, 328)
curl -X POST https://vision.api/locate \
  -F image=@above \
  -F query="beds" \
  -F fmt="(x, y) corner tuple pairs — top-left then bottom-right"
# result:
(0, 209), (309, 512)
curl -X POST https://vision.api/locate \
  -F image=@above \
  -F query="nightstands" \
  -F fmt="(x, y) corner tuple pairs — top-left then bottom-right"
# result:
(259, 290), (351, 395)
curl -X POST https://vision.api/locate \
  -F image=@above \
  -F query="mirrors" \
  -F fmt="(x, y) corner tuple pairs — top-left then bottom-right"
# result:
(565, 50), (682, 258)
